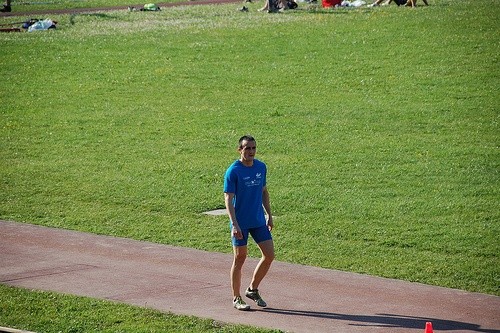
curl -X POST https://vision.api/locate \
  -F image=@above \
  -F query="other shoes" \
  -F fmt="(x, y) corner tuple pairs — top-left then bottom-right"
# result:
(382, 2), (390, 6)
(0, 5), (12, 12)
(367, 3), (379, 8)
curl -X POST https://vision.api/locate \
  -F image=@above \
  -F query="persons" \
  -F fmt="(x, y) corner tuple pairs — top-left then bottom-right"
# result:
(257, 0), (428, 12)
(222, 134), (276, 309)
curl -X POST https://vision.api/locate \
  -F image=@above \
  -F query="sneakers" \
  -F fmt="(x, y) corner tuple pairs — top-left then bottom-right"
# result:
(245, 287), (267, 307)
(232, 296), (251, 311)
